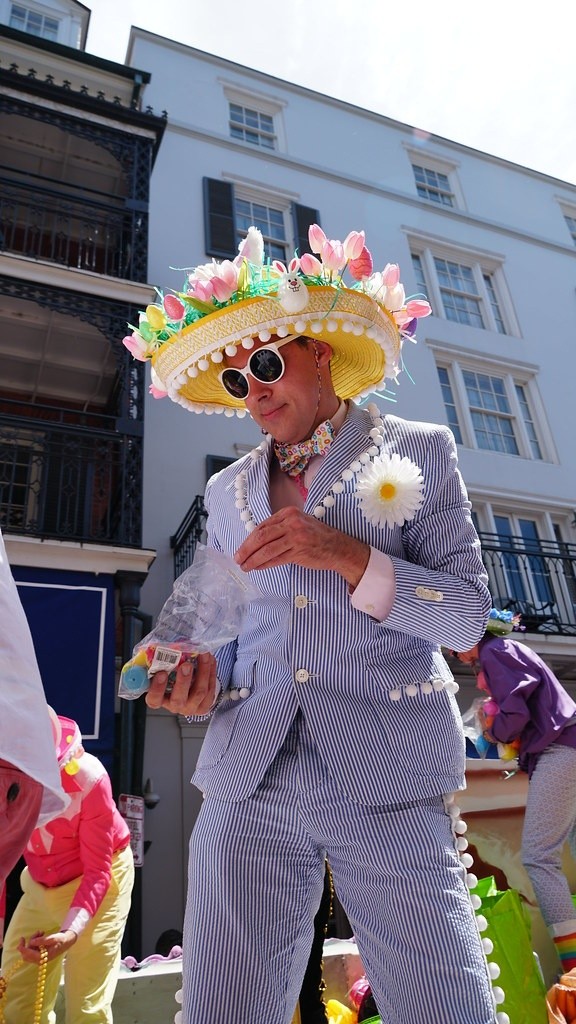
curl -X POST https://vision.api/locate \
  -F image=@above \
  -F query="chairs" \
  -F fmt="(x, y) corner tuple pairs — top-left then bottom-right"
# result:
(494, 598), (563, 633)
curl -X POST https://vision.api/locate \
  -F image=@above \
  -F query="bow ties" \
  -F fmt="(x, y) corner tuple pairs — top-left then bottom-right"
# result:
(274, 419), (337, 480)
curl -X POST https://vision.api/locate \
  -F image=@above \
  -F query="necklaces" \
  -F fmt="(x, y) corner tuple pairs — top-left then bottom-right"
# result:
(0, 944), (48, 1024)
(234, 404), (386, 534)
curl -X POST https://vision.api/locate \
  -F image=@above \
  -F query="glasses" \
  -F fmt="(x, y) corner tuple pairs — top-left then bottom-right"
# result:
(215, 333), (302, 401)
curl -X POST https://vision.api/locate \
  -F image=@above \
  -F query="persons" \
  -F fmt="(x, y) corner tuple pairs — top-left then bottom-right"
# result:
(118, 221), (513, 1024)
(0, 530), (134, 1024)
(442, 606), (576, 972)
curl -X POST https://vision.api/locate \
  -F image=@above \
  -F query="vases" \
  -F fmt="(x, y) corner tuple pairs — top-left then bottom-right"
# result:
(487, 619), (513, 635)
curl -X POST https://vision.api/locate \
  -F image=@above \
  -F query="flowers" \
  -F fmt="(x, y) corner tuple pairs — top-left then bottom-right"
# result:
(352, 454), (424, 530)
(489, 608), (526, 631)
(124, 223), (434, 399)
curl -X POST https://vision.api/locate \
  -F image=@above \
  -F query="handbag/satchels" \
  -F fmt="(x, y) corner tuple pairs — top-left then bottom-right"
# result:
(468, 875), (549, 1024)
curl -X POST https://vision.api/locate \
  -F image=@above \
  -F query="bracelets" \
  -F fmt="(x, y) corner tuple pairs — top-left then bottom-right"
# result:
(482, 731), (499, 744)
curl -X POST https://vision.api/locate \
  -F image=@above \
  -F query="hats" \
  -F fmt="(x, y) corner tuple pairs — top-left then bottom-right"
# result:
(123, 223), (431, 418)
(48, 704), (84, 772)
(449, 608), (525, 658)
